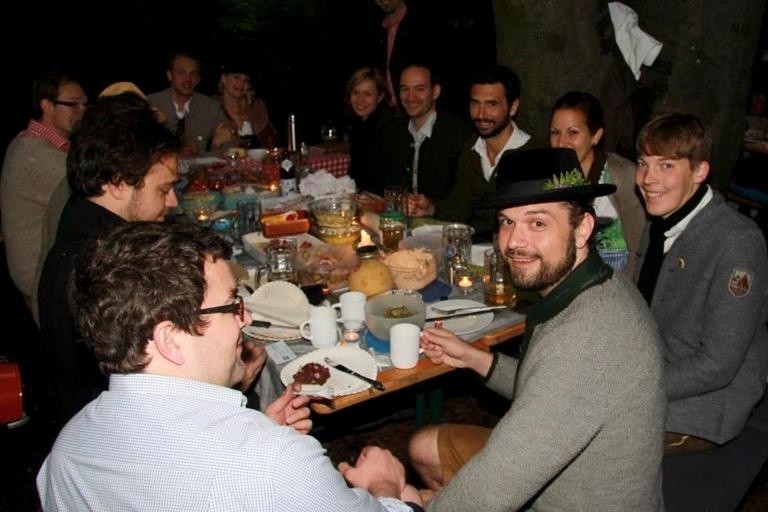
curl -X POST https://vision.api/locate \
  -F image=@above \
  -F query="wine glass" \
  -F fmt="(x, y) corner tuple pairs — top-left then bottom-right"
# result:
(321, 125), (338, 154)
(193, 192), (210, 230)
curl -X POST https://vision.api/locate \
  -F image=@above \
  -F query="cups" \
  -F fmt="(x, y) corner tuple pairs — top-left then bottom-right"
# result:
(382, 184), (408, 219)
(301, 305), (338, 347)
(253, 238), (299, 293)
(333, 291), (366, 332)
(236, 197), (262, 239)
(389, 323), (426, 370)
(442, 223), (474, 278)
(483, 248), (518, 311)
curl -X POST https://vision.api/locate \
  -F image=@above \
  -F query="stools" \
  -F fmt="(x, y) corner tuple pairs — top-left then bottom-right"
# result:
(719, 147), (767, 217)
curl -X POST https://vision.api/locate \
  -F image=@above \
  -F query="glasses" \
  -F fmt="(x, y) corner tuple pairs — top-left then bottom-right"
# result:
(179, 294), (245, 322)
(52, 99), (88, 109)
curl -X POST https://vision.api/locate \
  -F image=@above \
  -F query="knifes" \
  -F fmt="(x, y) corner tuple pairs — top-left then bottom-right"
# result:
(324, 355), (384, 392)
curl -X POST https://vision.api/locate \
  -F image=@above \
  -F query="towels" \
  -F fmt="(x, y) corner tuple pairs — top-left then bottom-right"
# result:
(608, 2), (664, 82)
(297, 170), (356, 201)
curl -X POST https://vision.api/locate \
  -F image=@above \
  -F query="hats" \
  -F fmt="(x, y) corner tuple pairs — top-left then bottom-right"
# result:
(461, 145), (618, 211)
(97, 81), (168, 123)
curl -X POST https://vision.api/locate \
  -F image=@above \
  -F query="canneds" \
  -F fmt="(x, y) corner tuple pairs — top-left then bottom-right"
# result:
(379, 212), (407, 254)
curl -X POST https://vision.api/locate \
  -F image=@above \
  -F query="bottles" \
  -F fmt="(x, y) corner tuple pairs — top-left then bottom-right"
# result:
(286, 112), (296, 154)
(278, 152), (298, 197)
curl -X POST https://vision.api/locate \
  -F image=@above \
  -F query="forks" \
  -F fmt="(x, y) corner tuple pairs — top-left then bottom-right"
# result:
(430, 305), (506, 314)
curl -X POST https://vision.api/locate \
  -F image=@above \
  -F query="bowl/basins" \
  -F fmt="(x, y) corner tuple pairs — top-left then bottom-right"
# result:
(317, 225), (366, 251)
(312, 198), (358, 226)
(366, 289), (425, 343)
(247, 148), (269, 161)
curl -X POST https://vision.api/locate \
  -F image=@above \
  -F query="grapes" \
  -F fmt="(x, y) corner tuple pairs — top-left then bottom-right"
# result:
(385, 305), (411, 318)
(330, 275), (337, 283)
(312, 274), (321, 281)
(302, 251), (309, 260)
(316, 249), (324, 257)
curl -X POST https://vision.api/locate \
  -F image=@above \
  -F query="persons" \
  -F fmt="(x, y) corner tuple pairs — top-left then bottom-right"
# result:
(360, 2), (446, 92)
(2, 46), (765, 512)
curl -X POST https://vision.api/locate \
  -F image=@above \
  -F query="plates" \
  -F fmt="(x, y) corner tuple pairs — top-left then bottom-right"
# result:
(240, 281), (308, 343)
(241, 227), (325, 263)
(414, 221), (474, 245)
(422, 299), (495, 335)
(280, 348), (378, 397)
(469, 243), (494, 268)
